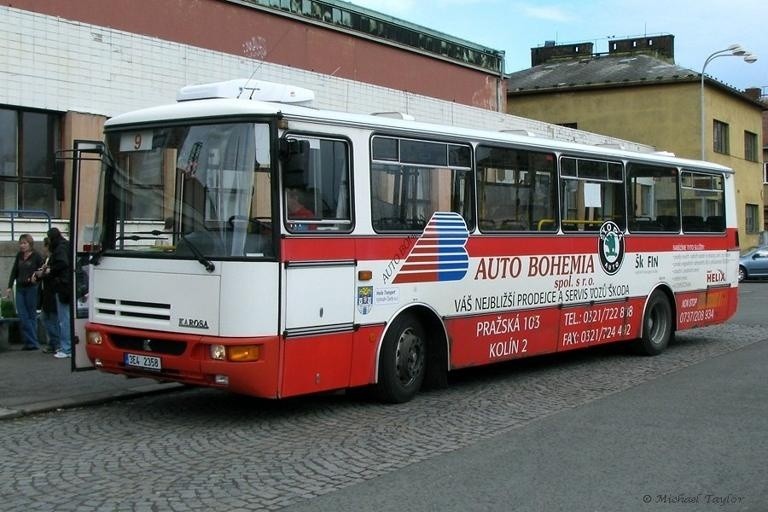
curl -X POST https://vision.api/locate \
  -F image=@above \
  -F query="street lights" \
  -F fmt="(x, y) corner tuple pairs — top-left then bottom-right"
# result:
(701, 43), (759, 161)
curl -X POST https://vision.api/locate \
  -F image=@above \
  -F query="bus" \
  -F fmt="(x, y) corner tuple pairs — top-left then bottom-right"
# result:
(52, 78), (740, 404)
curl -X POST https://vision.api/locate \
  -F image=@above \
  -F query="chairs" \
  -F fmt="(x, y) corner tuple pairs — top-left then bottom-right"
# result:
(373, 214), (726, 233)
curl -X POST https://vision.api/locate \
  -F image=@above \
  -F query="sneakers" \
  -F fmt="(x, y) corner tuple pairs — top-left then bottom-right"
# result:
(20, 343), (73, 359)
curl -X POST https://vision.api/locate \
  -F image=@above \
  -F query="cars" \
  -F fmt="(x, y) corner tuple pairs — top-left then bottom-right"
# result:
(738, 246), (768, 283)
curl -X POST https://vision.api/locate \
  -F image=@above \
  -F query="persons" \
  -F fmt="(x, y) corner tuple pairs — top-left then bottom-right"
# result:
(43, 227), (71, 358)
(283, 186), (318, 233)
(153, 215), (173, 248)
(30, 236), (60, 354)
(5, 234), (44, 352)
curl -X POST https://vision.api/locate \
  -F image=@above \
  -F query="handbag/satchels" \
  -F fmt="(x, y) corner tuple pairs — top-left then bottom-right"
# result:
(59, 266), (89, 306)
